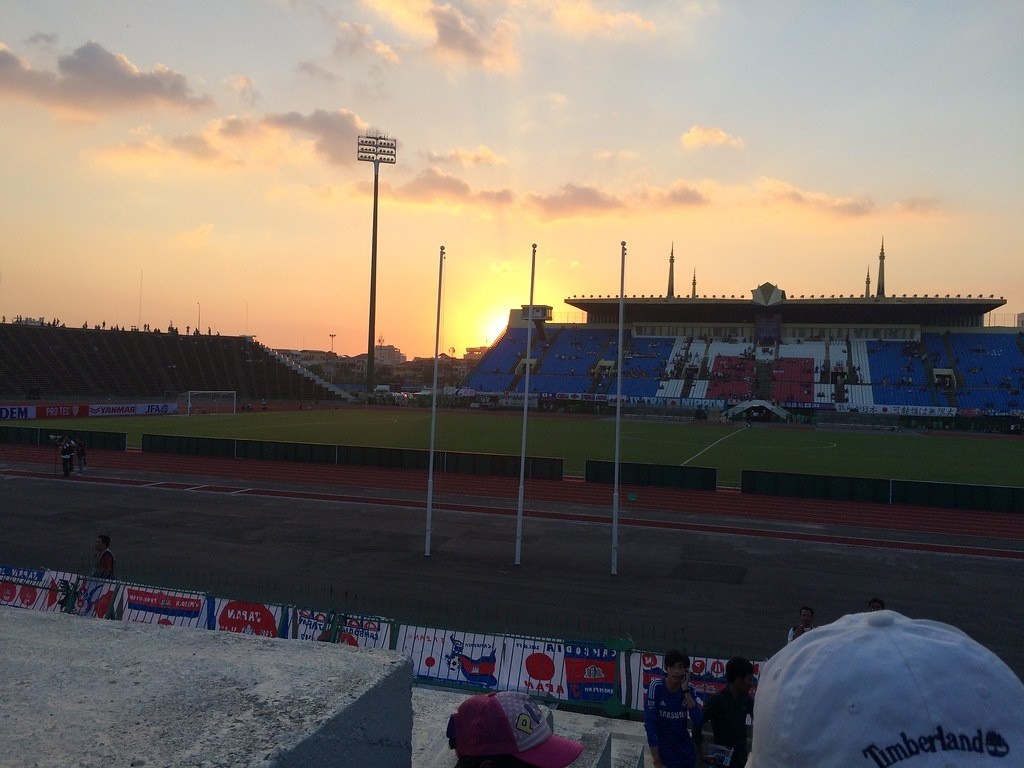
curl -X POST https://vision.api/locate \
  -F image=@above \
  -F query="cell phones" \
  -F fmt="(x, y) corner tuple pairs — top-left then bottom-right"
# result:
(682, 674), (686, 681)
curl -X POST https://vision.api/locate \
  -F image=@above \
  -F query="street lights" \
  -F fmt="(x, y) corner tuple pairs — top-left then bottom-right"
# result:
(329, 333), (336, 390)
(197, 301), (201, 331)
(378, 335), (385, 385)
(448, 346), (457, 387)
(353, 130), (397, 407)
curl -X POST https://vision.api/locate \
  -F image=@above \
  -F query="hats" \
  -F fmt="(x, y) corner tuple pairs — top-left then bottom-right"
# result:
(744, 609), (1024, 768)
(446, 690), (583, 768)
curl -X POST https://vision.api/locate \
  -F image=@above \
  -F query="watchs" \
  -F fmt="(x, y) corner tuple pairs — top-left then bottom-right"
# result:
(682, 687), (691, 694)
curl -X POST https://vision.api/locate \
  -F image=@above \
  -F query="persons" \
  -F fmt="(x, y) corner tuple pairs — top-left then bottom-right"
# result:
(0, 286), (1024, 431)
(446, 692), (585, 768)
(697, 656), (755, 768)
(90, 534), (116, 580)
(787, 606), (817, 644)
(59, 434), (86, 479)
(746, 609), (1024, 767)
(868, 597), (885, 612)
(643, 649), (704, 768)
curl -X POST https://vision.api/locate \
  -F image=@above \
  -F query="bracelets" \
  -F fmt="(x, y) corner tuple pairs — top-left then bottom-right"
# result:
(653, 758), (661, 764)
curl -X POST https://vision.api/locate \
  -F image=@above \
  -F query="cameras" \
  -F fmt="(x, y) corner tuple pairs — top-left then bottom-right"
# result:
(48, 435), (64, 442)
(800, 625), (811, 632)
